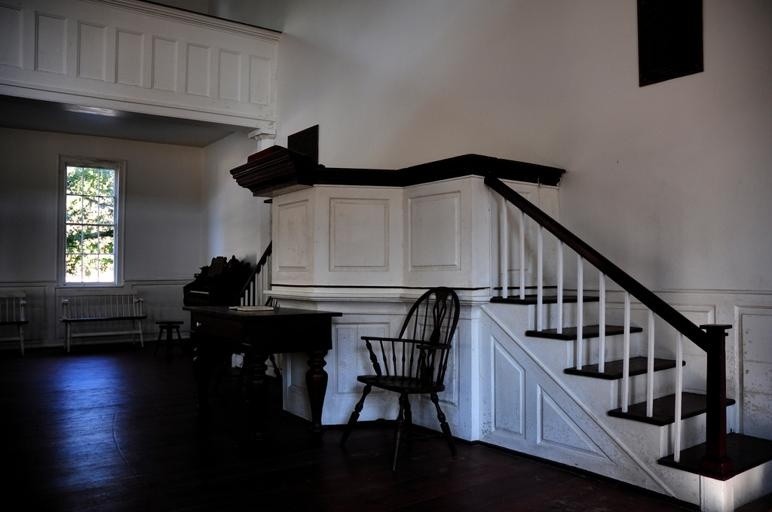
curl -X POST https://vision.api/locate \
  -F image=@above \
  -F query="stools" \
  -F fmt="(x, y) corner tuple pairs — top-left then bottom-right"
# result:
(153, 321), (184, 356)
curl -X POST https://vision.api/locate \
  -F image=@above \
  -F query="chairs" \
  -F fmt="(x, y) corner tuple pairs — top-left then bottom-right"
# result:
(337, 287), (461, 471)
(1, 290), (28, 356)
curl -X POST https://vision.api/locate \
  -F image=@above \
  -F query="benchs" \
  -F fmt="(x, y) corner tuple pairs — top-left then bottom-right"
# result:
(59, 292), (146, 352)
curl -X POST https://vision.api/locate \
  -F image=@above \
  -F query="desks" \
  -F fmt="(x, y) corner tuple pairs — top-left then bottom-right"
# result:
(182, 305), (343, 434)
